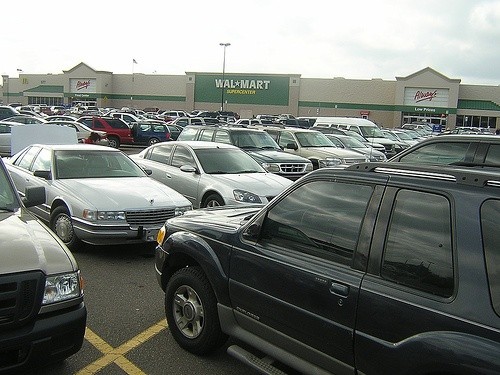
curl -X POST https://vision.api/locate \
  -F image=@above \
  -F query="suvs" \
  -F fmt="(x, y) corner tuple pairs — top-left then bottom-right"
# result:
(154, 161), (500, 375)
(0, 102), (500, 181)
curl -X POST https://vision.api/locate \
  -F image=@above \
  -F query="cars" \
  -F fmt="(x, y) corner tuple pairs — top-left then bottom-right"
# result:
(0, 125), (194, 248)
(127, 140), (295, 209)
(0, 156), (89, 375)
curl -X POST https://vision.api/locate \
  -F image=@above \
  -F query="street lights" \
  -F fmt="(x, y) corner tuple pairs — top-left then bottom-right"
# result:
(219, 42), (231, 111)
